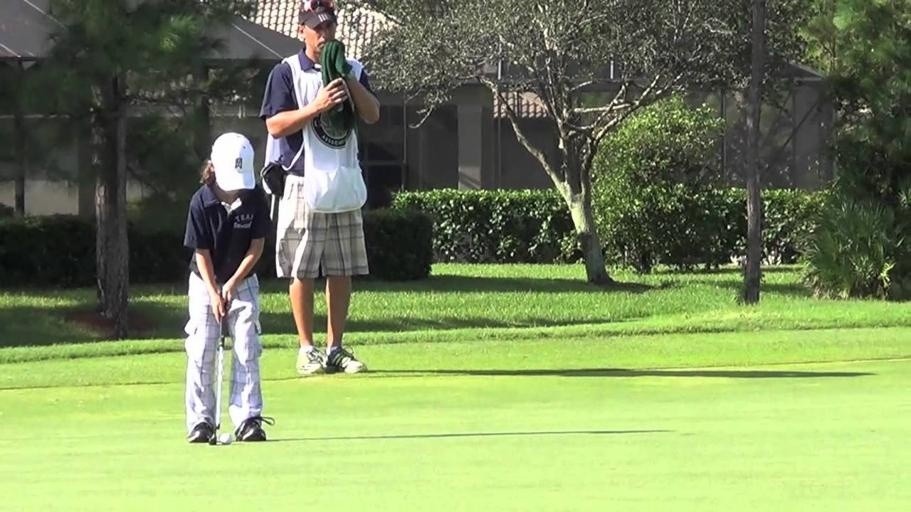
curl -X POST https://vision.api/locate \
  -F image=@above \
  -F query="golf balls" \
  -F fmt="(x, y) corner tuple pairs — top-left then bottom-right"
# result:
(221, 433), (232, 445)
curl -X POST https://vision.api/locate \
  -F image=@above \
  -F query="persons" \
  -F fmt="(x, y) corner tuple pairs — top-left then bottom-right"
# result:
(259, 1), (384, 374)
(180, 131), (276, 444)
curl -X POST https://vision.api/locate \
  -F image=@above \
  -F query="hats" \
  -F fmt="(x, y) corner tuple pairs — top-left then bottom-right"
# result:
(209, 131), (258, 193)
(298, 5), (339, 28)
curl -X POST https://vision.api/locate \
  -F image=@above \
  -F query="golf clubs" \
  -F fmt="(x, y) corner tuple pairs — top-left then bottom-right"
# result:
(208, 287), (223, 444)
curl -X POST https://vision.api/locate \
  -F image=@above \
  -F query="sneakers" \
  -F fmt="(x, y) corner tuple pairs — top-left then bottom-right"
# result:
(294, 348), (326, 376)
(236, 418), (268, 442)
(325, 348), (368, 374)
(186, 422), (215, 443)
